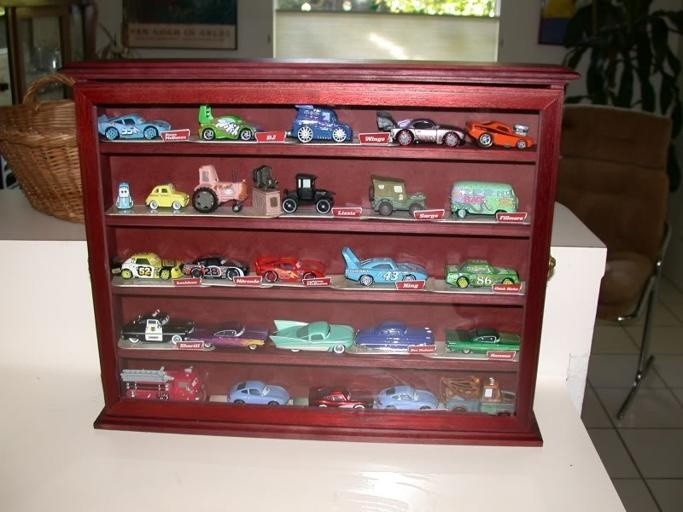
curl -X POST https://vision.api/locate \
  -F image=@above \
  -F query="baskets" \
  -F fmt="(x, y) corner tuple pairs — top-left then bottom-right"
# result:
(0, 74), (87, 223)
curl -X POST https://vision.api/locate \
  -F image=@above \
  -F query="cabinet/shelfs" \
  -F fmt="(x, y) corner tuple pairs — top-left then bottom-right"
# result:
(65, 60), (579, 447)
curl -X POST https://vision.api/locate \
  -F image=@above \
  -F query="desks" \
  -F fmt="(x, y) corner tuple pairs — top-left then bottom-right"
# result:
(0, 187), (628, 512)
(0, 0), (97, 104)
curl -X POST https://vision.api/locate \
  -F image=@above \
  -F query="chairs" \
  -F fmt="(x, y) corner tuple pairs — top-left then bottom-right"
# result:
(556, 104), (671, 420)
